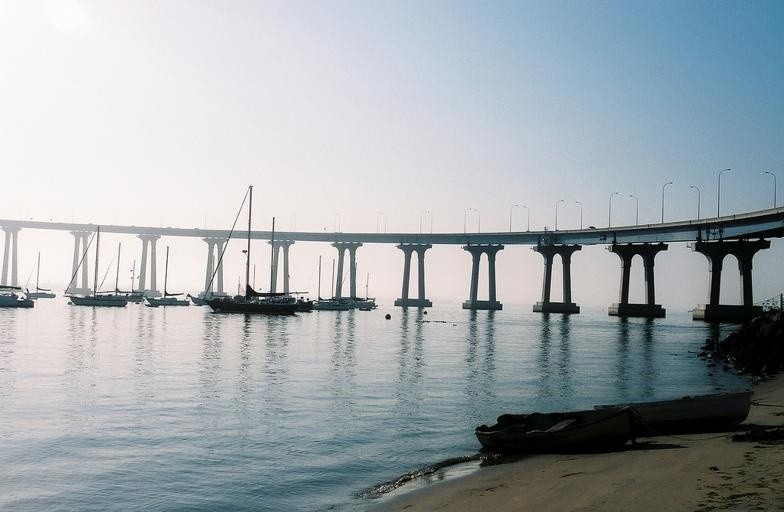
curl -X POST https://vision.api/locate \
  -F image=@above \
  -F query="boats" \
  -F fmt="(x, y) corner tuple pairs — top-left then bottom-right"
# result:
(314, 295), (378, 311)
(474, 403), (636, 457)
(593, 388), (755, 435)
(0, 285), (34, 308)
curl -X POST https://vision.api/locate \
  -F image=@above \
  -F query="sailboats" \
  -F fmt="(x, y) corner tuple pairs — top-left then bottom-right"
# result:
(23, 251), (57, 299)
(190, 183), (314, 315)
(145, 246), (190, 307)
(64, 225), (127, 307)
(84, 241), (145, 302)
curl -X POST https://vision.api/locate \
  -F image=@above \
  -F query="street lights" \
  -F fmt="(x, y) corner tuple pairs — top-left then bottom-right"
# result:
(661, 181), (673, 224)
(332, 199), (584, 233)
(765, 171), (776, 208)
(717, 168), (731, 218)
(608, 192), (618, 228)
(690, 186), (700, 220)
(630, 195), (638, 226)
